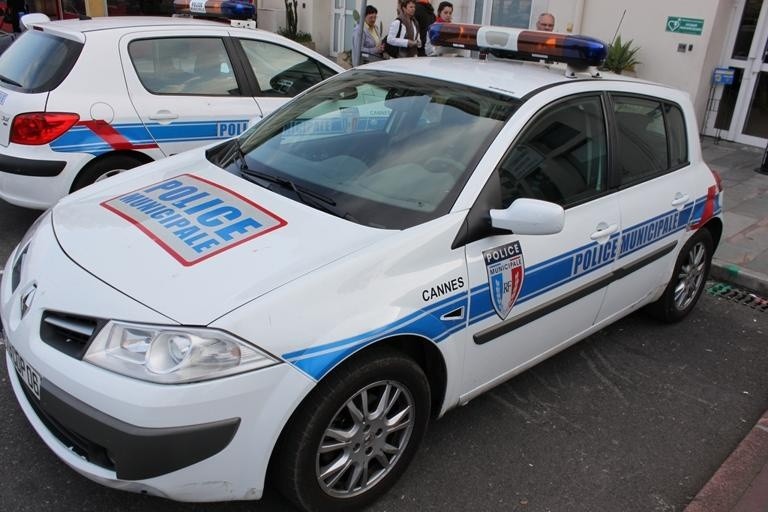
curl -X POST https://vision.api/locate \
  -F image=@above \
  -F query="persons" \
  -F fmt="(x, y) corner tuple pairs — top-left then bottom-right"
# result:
(535, 12), (556, 32)
(386, 0), (424, 58)
(398, 0), (436, 57)
(350, 3), (386, 67)
(424, 0), (457, 57)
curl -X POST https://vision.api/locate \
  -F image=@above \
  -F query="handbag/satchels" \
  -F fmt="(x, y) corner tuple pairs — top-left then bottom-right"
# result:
(381, 34), (400, 58)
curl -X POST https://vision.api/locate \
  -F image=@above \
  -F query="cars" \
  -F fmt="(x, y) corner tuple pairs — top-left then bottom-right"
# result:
(0, 2), (385, 214)
(1, 17), (721, 512)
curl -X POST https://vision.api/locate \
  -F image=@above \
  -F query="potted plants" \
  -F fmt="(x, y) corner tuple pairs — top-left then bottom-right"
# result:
(276, 0), (315, 50)
